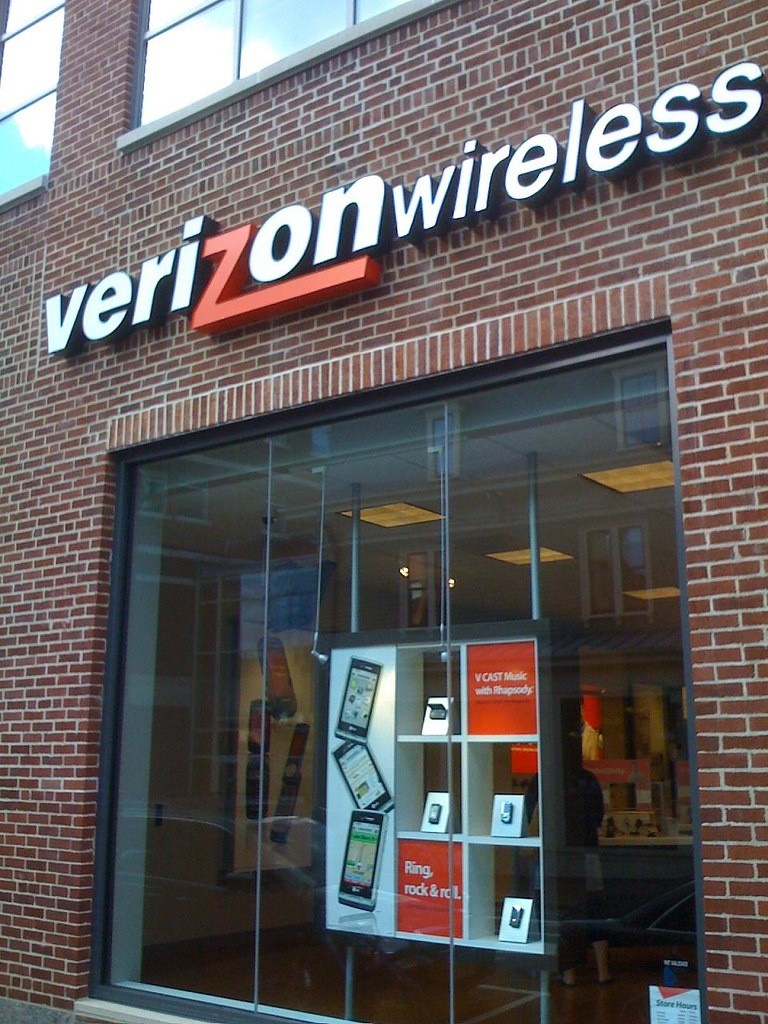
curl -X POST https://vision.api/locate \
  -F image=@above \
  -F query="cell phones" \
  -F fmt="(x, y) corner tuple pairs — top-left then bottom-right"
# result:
(332, 656), (395, 914)
(246, 636), (310, 843)
(429, 804), (441, 825)
(510, 906), (523, 928)
(501, 802), (513, 824)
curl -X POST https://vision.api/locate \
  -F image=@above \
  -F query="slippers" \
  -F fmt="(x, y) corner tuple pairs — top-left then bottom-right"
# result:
(559, 974), (576, 987)
(600, 976), (615, 985)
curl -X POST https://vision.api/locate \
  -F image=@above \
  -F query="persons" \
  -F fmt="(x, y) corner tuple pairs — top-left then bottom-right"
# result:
(519, 737), (613, 984)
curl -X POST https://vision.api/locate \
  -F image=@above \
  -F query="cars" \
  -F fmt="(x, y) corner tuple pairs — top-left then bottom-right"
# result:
(493, 880), (696, 981)
(108, 809), (395, 983)
